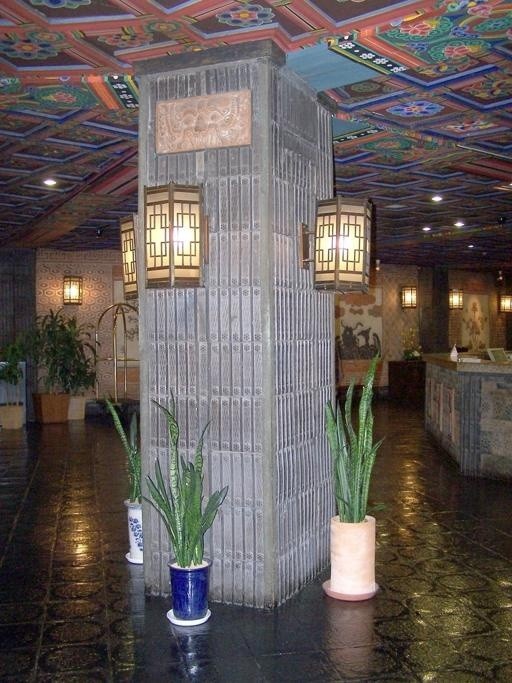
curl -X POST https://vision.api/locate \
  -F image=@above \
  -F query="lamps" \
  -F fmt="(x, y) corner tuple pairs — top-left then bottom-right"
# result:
(401, 286), (418, 308)
(499, 294), (512, 312)
(118, 182), (209, 301)
(301, 195), (371, 293)
(63, 278), (83, 303)
(448, 289), (464, 311)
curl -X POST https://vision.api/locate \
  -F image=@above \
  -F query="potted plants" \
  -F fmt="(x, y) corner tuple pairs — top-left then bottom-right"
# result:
(0, 308), (101, 431)
(103, 387), (228, 626)
(321, 347), (387, 602)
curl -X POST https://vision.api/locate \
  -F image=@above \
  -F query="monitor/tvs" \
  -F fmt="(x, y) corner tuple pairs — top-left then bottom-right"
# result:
(487, 348), (509, 362)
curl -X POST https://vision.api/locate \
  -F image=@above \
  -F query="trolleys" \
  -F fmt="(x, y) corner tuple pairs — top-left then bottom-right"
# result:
(86, 301), (140, 428)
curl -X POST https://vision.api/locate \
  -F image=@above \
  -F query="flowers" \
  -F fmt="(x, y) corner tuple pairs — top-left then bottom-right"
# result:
(400, 325), (422, 350)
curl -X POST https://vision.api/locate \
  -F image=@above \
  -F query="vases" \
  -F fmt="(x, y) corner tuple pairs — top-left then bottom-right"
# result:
(404, 350), (421, 361)
